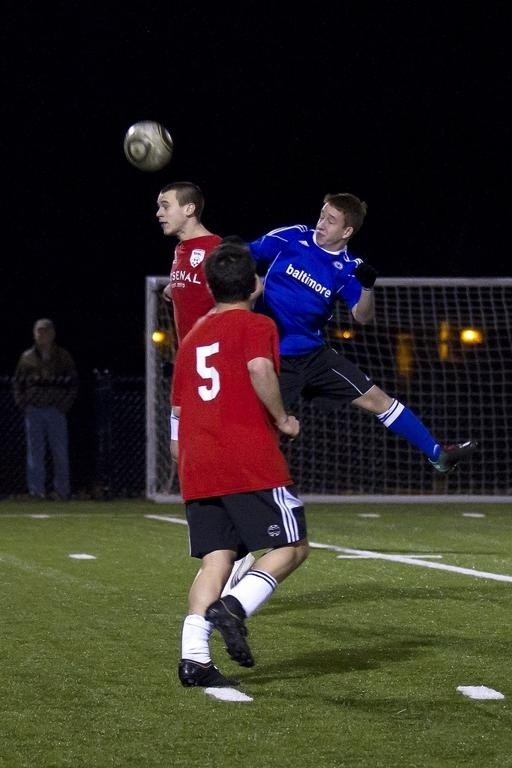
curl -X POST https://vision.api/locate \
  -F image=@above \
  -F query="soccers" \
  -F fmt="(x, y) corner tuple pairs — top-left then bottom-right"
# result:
(124, 121), (173, 171)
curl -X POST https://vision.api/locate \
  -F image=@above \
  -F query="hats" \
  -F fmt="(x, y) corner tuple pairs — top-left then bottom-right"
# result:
(35, 318), (54, 330)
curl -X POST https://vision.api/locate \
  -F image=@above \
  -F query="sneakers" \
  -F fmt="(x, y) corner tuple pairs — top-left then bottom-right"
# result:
(179, 660), (239, 686)
(204, 596), (255, 667)
(220, 553), (255, 599)
(428, 441), (478, 473)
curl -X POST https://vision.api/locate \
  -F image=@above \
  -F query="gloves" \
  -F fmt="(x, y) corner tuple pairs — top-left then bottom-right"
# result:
(353, 263), (376, 288)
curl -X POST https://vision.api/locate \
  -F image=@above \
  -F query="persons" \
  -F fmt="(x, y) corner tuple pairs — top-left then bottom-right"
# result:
(12, 319), (81, 501)
(166, 239), (312, 682)
(251, 194), (480, 474)
(155, 178), (264, 351)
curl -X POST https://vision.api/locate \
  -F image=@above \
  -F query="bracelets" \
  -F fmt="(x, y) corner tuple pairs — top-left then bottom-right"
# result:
(169, 410), (180, 441)
